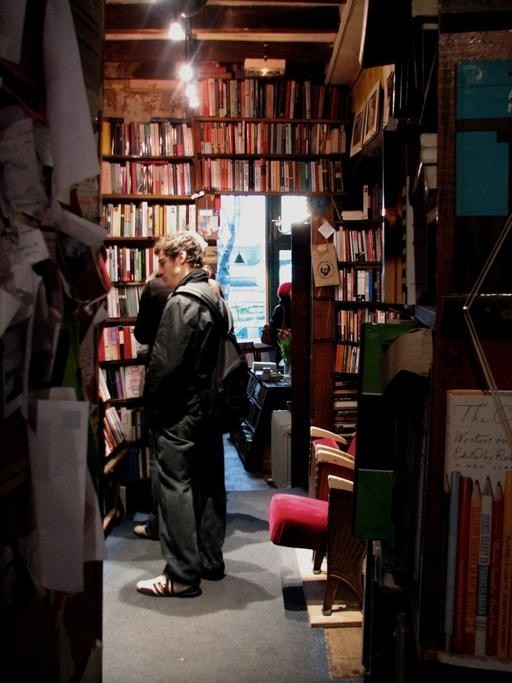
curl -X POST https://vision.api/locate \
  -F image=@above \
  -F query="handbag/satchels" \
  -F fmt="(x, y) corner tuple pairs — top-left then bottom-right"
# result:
(261, 313), (278, 346)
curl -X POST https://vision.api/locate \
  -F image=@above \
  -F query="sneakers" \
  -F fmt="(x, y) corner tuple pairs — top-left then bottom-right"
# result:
(136, 574), (202, 597)
(134, 525), (147, 536)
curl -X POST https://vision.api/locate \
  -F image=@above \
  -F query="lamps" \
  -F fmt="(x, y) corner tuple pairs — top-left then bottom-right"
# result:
(158, 0), (208, 107)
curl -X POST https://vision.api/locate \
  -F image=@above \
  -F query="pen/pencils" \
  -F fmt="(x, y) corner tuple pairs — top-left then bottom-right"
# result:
(442, 469), (512, 662)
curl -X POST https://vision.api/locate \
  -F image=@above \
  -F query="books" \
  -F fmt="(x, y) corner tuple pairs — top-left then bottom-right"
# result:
(425, 203), (438, 227)
(422, 163), (438, 211)
(326, 176), (425, 441)
(216, 74), (352, 194)
(435, 389), (512, 673)
(198, 78), (216, 116)
(102, 119), (216, 238)
(453, 58), (512, 123)
(453, 129), (511, 218)
(418, 131), (439, 147)
(411, 146), (438, 197)
(103, 246), (160, 512)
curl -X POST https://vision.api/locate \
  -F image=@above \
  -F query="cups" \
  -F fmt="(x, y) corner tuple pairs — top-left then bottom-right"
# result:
(262, 367), (270, 379)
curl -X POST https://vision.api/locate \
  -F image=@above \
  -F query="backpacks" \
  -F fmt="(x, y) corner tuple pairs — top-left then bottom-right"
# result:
(176, 285), (249, 434)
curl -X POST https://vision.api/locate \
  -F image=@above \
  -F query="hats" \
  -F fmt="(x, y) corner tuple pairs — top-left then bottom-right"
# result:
(278, 282), (292, 297)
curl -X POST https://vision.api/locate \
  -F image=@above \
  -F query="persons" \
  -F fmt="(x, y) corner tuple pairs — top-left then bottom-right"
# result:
(129, 228), (225, 540)
(135, 228), (238, 598)
(266, 281), (292, 369)
(126, 94), (144, 113)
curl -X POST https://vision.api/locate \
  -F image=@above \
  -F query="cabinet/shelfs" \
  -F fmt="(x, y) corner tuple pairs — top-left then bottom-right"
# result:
(102, 76), (406, 541)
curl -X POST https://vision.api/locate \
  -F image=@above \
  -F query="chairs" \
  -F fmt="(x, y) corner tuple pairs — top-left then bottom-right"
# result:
(267, 449), (367, 615)
